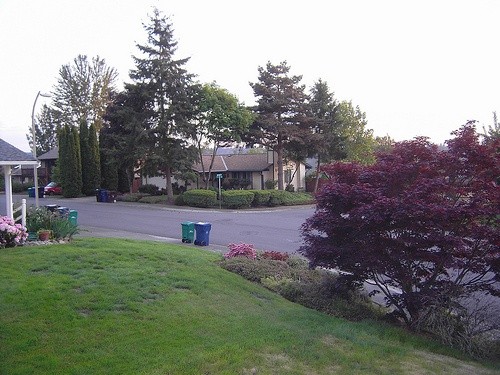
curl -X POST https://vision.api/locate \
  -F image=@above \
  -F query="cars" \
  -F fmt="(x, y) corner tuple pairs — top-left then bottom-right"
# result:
(44, 182), (63, 196)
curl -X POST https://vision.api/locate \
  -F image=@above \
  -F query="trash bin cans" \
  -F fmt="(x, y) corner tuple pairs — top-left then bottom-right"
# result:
(180, 221), (195, 243)
(28, 186), (44, 198)
(95, 190), (115, 203)
(64, 210), (77, 226)
(194, 221), (211, 246)
(46, 204), (57, 214)
(56, 207), (69, 219)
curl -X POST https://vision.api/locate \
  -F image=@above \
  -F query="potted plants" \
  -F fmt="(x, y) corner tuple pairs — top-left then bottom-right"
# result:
(36, 227), (57, 242)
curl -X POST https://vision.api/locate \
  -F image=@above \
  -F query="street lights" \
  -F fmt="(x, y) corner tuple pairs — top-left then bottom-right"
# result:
(31, 90), (52, 206)
